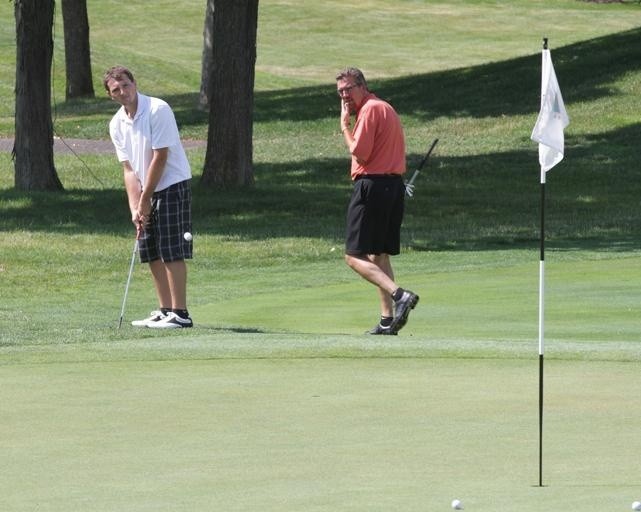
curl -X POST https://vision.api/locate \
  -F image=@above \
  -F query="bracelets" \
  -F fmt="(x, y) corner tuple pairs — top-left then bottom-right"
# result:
(342, 128), (353, 134)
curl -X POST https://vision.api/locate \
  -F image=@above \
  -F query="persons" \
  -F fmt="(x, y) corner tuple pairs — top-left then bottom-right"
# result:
(102, 66), (195, 329)
(337, 68), (420, 336)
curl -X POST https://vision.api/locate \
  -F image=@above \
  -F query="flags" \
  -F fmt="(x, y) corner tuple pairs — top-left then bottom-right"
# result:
(530, 46), (571, 186)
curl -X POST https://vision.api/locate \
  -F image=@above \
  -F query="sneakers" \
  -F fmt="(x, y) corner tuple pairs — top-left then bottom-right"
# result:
(363, 321), (398, 336)
(389, 290), (419, 331)
(132, 310), (169, 328)
(144, 311), (193, 329)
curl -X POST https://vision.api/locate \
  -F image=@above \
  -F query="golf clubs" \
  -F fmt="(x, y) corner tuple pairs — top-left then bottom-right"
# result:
(109, 215), (141, 328)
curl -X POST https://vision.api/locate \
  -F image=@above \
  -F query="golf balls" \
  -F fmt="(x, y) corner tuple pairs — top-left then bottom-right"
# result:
(184, 232), (192, 241)
(453, 500), (462, 509)
(632, 502), (641, 510)
